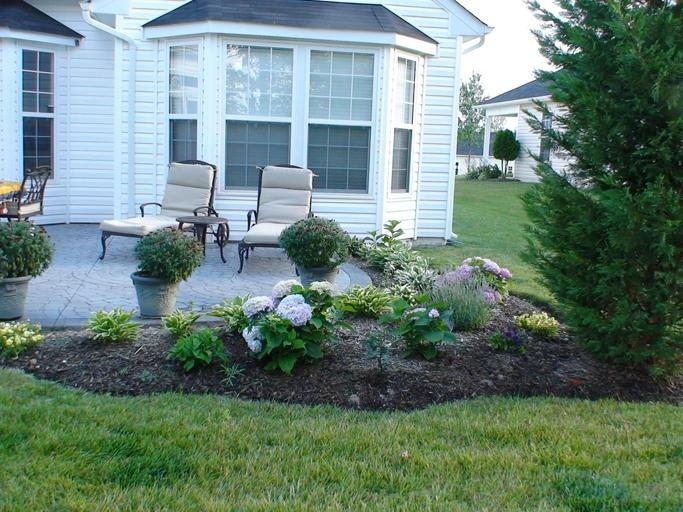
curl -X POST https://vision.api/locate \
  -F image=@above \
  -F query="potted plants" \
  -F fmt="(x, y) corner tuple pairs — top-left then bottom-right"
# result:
(0, 219), (56, 320)
(130, 230), (204, 317)
(278, 216), (353, 290)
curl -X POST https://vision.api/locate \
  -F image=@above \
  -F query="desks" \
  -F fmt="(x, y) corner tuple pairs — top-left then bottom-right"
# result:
(176, 216), (228, 263)
(0, 180), (23, 202)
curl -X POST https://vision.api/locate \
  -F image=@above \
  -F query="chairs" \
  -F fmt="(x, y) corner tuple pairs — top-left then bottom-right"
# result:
(99, 160), (216, 260)
(0, 165), (52, 221)
(238, 164), (317, 275)
